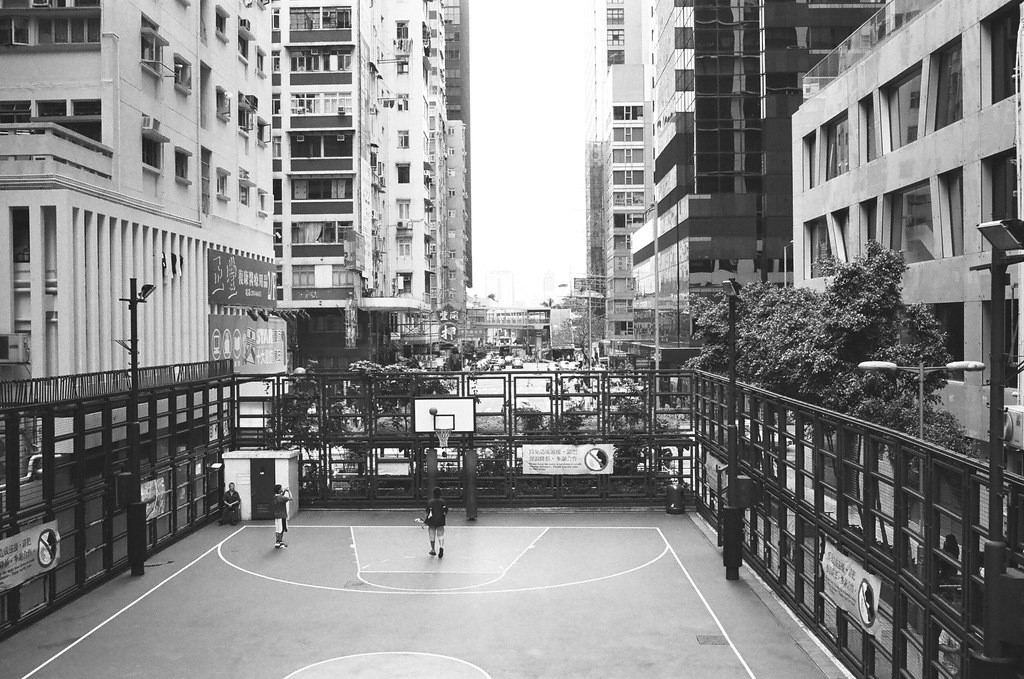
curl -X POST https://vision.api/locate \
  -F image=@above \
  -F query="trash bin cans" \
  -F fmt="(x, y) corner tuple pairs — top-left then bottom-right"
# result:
(665, 483), (687, 515)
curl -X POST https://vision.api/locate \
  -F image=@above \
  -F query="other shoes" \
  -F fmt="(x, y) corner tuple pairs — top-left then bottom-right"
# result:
(274, 544), (288, 549)
(438, 547), (443, 559)
(429, 550), (436, 555)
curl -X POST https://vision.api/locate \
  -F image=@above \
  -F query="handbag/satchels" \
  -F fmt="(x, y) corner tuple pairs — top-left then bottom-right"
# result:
(428, 507), (432, 519)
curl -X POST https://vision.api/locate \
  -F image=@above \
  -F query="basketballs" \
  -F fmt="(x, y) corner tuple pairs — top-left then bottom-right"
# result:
(428, 407), (439, 415)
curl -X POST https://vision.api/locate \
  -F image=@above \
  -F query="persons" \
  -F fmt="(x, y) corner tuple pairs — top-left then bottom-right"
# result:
(422, 488), (449, 558)
(272, 485), (293, 548)
(217, 482), (240, 526)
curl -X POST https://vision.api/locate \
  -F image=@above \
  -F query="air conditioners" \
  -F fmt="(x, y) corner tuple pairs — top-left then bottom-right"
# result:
(397, 218), (413, 230)
(0, 332), (28, 363)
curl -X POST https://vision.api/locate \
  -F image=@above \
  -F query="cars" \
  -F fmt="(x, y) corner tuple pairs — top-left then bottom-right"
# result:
(476, 351), (550, 371)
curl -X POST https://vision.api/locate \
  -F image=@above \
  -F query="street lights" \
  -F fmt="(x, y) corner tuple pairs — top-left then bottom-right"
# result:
(118, 277), (156, 580)
(858, 360), (987, 540)
(966, 216), (1024, 679)
(560, 283), (591, 370)
(718, 279), (750, 576)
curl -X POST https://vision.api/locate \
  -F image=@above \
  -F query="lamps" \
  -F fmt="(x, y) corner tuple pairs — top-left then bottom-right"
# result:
(974, 219), (1024, 253)
(721, 278), (742, 298)
(138, 283), (156, 303)
